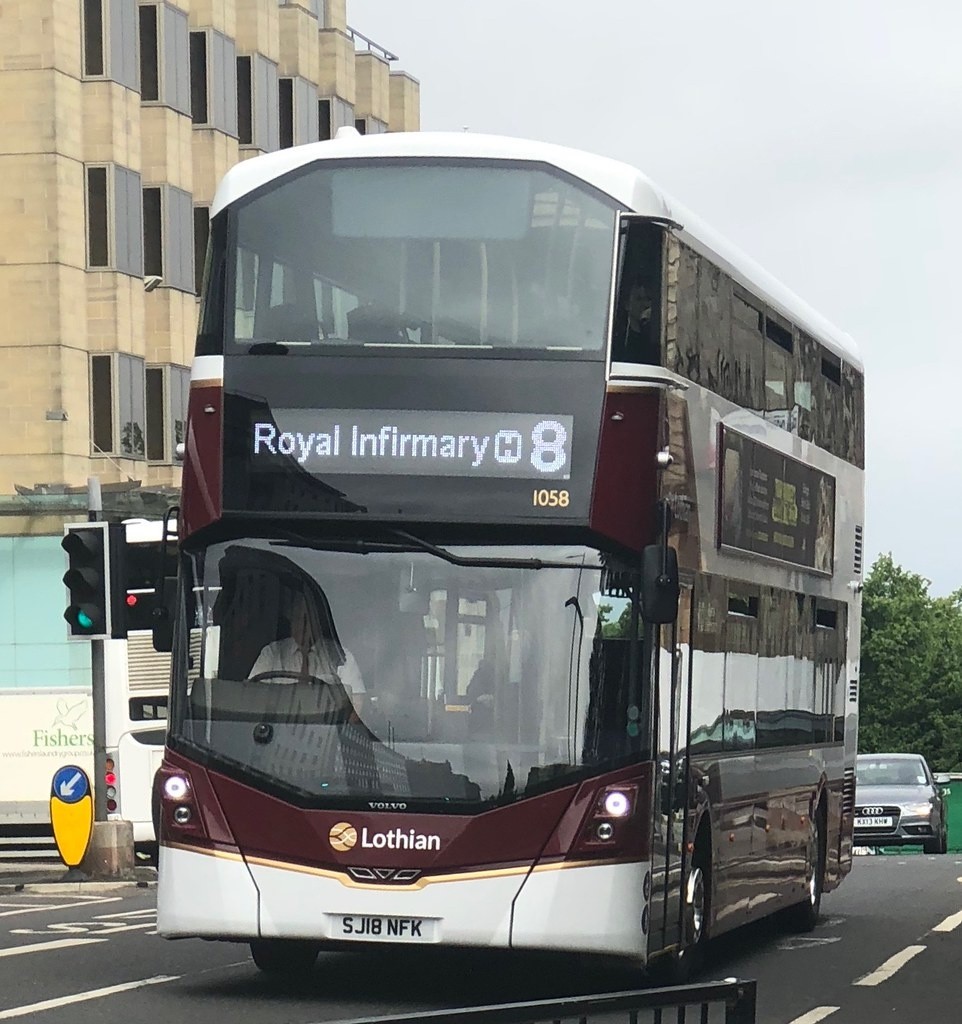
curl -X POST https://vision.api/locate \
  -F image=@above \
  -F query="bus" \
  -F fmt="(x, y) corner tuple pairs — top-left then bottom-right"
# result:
(144, 127), (871, 975)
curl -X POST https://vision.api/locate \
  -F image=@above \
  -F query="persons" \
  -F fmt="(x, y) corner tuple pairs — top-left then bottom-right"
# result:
(247, 599), (367, 723)
(898, 766), (920, 782)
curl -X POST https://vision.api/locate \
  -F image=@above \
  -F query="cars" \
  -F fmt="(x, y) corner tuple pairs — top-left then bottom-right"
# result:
(853, 752), (952, 855)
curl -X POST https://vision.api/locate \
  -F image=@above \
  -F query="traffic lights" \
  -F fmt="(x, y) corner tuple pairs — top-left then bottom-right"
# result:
(60, 519), (112, 642)
(109, 519), (157, 639)
(107, 750), (120, 814)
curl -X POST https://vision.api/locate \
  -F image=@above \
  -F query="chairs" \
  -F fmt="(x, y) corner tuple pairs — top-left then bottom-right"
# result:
(259, 300), (412, 345)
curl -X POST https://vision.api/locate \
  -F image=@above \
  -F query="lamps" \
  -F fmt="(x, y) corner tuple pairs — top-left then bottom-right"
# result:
(143, 274), (163, 292)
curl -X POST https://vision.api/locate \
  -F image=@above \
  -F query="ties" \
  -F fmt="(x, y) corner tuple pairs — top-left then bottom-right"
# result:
(299, 647), (312, 681)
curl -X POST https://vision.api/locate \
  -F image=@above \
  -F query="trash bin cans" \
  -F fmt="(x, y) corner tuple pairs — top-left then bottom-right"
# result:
(933, 773), (962, 853)
